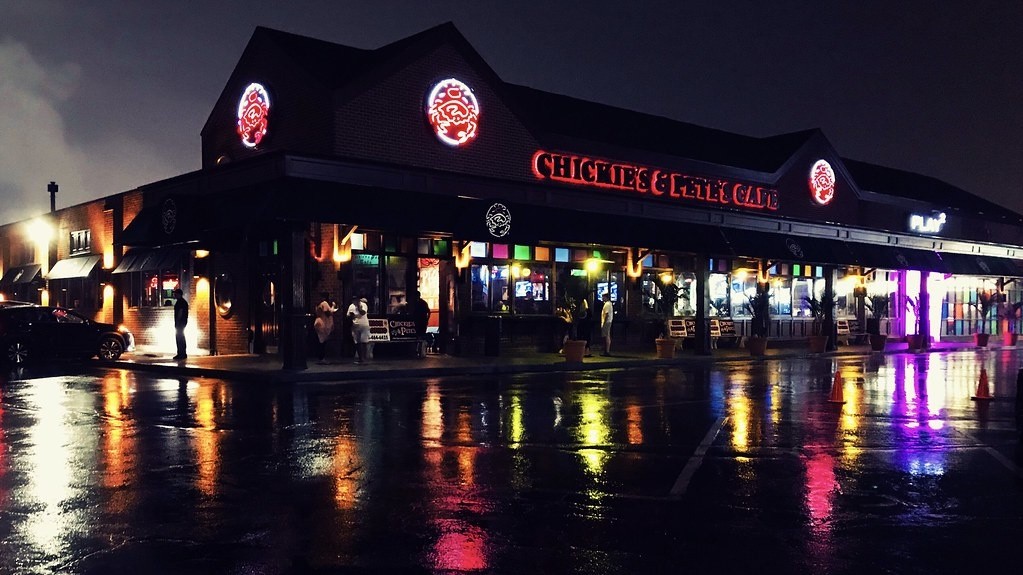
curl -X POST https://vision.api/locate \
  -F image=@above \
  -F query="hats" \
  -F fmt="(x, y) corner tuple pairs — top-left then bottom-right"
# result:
(172, 289), (183, 295)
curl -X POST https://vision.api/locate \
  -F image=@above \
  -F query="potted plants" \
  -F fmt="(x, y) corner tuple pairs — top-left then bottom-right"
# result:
(966, 288), (1000, 347)
(860, 296), (892, 351)
(1000, 301), (1019, 347)
(641, 272), (690, 358)
(558, 295), (588, 362)
(800, 294), (830, 353)
(743, 293), (772, 356)
(905, 295), (924, 349)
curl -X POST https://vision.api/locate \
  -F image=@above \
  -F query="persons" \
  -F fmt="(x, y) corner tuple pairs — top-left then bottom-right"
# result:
(346, 296), (370, 365)
(576, 300), (591, 357)
(417, 291), (431, 333)
(173, 289), (188, 360)
(315, 290), (338, 365)
(556, 307), (571, 354)
(600, 294), (613, 357)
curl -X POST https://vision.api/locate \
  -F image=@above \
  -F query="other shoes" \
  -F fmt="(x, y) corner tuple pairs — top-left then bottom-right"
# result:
(600, 353), (609, 356)
(174, 354), (186, 360)
(316, 359), (330, 365)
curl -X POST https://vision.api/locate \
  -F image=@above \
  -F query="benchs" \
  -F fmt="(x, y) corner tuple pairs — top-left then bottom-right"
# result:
(682, 316), (746, 350)
(362, 315), (429, 358)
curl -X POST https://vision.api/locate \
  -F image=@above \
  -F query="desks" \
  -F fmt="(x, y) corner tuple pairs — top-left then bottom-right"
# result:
(485, 313), (553, 357)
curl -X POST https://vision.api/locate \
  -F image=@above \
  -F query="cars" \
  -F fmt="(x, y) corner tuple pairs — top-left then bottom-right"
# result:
(0, 306), (131, 366)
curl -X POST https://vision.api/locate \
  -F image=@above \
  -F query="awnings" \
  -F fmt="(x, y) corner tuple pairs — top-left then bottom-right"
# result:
(46, 255), (101, 280)
(0, 265), (41, 289)
(111, 246), (181, 274)
